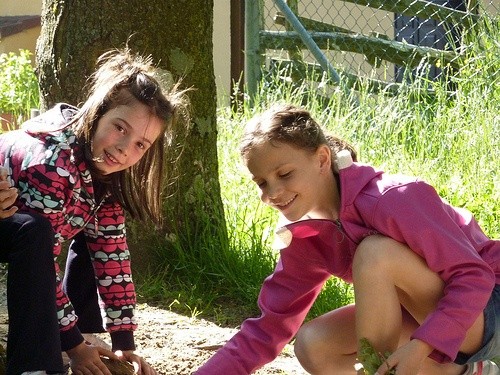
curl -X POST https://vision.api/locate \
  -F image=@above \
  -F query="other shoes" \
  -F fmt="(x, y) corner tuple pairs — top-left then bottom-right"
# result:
(461, 360), (498, 375)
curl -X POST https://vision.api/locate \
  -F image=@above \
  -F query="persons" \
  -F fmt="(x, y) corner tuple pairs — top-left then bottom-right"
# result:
(0, 32), (197, 375)
(189, 105), (500, 375)
